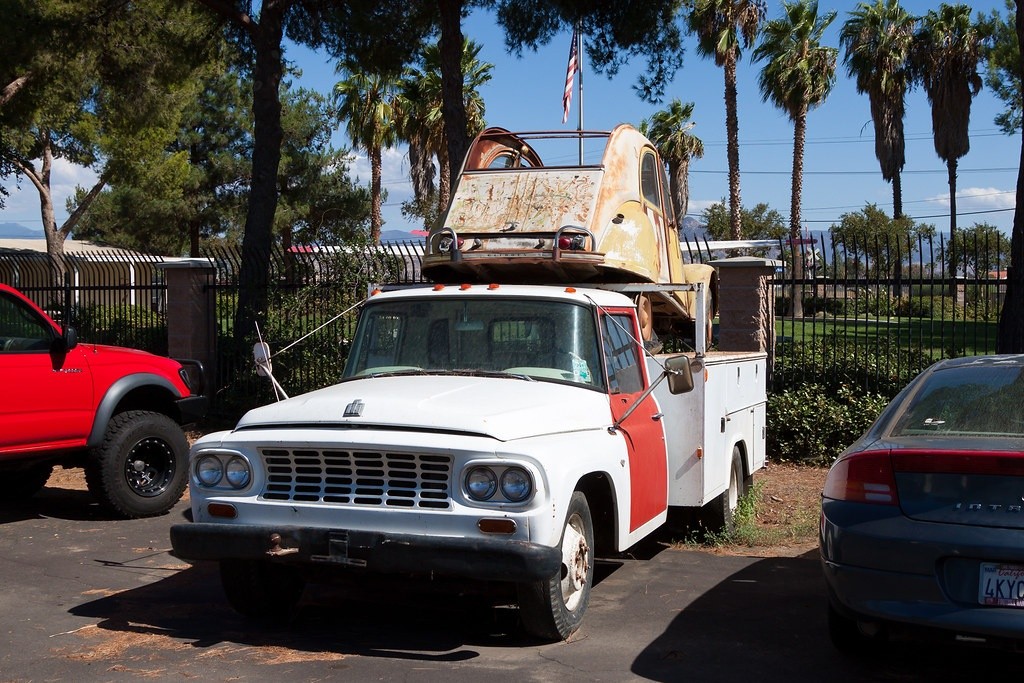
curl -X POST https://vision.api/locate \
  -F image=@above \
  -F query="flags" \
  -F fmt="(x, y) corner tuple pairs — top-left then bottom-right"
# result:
(562, 20), (582, 124)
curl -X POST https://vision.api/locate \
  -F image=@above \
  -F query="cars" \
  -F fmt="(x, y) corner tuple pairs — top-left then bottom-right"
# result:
(0, 282), (212, 521)
(817, 353), (1023, 660)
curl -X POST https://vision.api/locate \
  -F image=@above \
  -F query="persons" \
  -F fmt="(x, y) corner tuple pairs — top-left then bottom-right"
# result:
(802, 259), (813, 279)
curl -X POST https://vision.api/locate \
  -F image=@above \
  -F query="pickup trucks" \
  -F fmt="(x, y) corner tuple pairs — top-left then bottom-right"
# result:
(169, 281), (769, 642)
